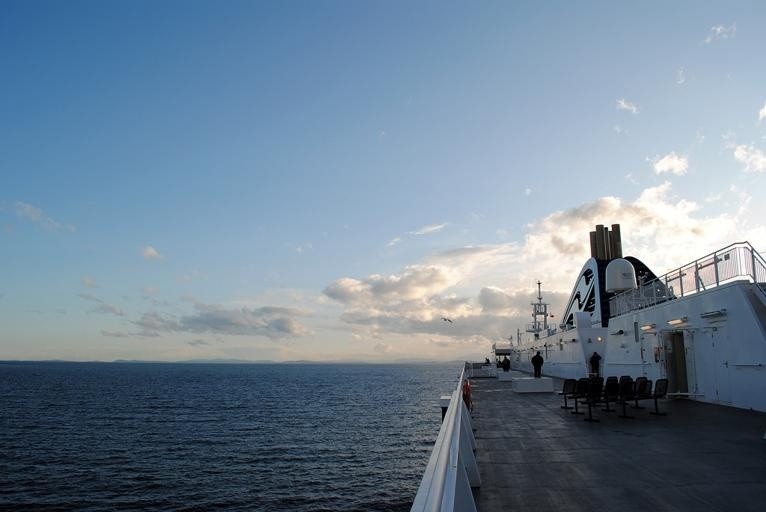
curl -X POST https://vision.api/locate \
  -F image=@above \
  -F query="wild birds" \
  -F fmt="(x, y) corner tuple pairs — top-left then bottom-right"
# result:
(441, 318), (453, 323)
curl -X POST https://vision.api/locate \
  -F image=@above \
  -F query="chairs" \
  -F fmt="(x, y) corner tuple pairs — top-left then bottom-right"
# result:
(557, 375), (669, 423)
(613, 286), (677, 317)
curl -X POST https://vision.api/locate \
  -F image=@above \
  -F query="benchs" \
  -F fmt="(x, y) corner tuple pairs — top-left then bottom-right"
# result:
(482, 365), (553, 392)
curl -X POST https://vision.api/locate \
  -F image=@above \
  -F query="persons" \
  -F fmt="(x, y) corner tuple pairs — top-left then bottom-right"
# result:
(530, 351), (544, 379)
(588, 351), (602, 378)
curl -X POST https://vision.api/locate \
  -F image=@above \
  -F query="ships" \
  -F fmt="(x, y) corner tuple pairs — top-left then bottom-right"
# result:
(410, 224), (766, 512)
